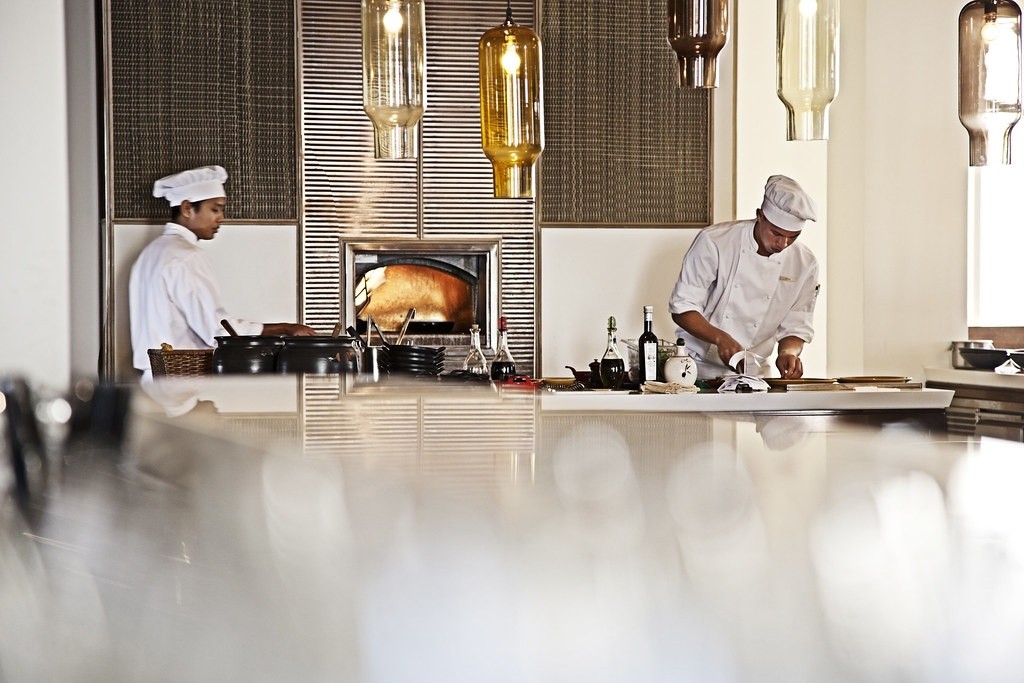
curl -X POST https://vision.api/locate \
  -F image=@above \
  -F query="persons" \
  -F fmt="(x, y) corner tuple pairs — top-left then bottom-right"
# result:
(129, 165), (318, 422)
(668, 175), (820, 380)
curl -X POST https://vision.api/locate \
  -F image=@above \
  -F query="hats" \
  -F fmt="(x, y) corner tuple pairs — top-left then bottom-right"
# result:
(151, 165), (229, 208)
(761, 174), (816, 232)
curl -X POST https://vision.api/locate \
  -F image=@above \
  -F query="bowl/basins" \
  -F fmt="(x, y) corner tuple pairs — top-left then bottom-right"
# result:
(958, 346), (1008, 369)
(947, 340), (998, 369)
(1010, 350), (1024, 370)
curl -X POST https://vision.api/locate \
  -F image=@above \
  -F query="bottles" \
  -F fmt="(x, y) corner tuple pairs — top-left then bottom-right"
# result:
(664, 338), (698, 386)
(638, 305), (658, 386)
(597, 316), (625, 387)
(462, 324), (488, 374)
(489, 316), (516, 383)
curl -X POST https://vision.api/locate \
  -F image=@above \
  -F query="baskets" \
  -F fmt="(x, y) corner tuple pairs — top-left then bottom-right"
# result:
(145, 348), (216, 382)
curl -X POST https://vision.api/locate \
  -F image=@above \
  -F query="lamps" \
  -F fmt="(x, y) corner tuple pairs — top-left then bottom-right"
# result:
(666, 0), (729, 90)
(478, 0), (545, 199)
(361, 0), (428, 160)
(958, 0), (1023, 165)
(777, 0), (842, 142)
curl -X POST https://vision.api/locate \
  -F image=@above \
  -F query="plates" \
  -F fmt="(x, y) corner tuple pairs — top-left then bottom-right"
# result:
(542, 377), (577, 384)
(837, 376), (913, 383)
(761, 379), (836, 389)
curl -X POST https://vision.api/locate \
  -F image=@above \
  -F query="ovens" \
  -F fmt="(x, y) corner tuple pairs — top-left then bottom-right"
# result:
(346, 242), (498, 358)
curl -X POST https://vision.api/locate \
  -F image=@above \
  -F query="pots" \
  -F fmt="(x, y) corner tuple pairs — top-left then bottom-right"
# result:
(380, 341), (493, 386)
(565, 365), (629, 388)
(212, 335), (277, 374)
(277, 335), (356, 375)
